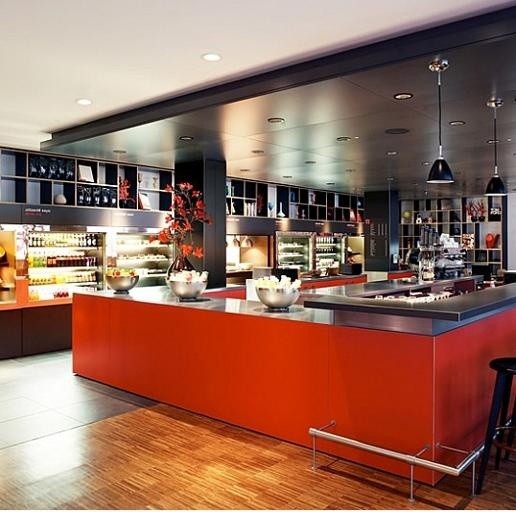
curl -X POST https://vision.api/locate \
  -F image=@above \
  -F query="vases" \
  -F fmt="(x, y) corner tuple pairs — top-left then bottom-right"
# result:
(119, 199), (128, 208)
(471, 217), (477, 222)
(168, 255), (195, 280)
(148, 182), (214, 259)
(485, 233), (495, 249)
(479, 216), (485, 222)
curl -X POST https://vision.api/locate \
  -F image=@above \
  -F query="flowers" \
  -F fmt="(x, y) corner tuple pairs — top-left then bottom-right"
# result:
(118, 178), (131, 199)
(465, 200), (478, 216)
(478, 199), (487, 216)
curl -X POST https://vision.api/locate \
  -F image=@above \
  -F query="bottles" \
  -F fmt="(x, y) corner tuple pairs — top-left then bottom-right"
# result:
(53, 290), (70, 299)
(29, 291), (42, 302)
(28, 232), (103, 286)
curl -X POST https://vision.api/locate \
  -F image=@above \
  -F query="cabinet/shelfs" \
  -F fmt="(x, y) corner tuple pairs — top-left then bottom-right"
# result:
(21, 303), (71, 357)
(274, 231), (315, 272)
(1, 223), (104, 303)
(0, 147), (174, 212)
(399, 196), (507, 274)
(106, 226), (174, 289)
(314, 235), (346, 272)
(224, 175), (364, 222)
(0, 308), (21, 360)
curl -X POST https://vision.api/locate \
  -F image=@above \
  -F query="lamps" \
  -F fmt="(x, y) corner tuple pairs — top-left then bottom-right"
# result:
(230, 235), (240, 249)
(484, 97), (508, 197)
(241, 236), (254, 248)
(425, 59), (455, 184)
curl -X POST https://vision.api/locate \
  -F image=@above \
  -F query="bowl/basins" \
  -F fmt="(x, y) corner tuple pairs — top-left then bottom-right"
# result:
(255, 287), (300, 311)
(168, 281), (207, 301)
(106, 276), (140, 295)
(240, 263), (252, 270)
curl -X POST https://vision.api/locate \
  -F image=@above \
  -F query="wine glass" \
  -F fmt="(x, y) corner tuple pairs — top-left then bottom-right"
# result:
(138, 173), (143, 188)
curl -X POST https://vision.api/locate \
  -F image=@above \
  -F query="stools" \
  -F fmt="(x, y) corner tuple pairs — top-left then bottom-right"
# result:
(476, 357), (515, 495)
